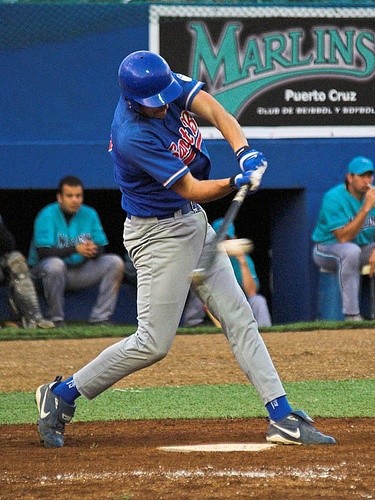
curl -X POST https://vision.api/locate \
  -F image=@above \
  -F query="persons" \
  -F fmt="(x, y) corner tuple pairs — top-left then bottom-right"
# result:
(34, 50), (336, 449)
(182, 218), (272, 329)
(0, 216), (55, 328)
(312, 156), (375, 321)
(29, 175), (125, 327)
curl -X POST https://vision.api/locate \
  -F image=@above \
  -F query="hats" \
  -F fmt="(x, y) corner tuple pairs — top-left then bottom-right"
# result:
(348, 157), (374, 175)
(212, 219), (235, 239)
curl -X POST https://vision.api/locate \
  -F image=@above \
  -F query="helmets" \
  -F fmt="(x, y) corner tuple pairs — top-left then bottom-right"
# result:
(118, 50), (183, 112)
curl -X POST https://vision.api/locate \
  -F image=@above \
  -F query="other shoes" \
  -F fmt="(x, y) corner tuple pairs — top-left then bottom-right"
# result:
(344, 314), (362, 322)
(54, 320), (67, 328)
(17, 319), (55, 329)
(97, 320), (114, 328)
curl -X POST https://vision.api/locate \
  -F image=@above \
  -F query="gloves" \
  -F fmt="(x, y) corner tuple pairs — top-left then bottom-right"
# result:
(235, 146), (268, 176)
(230, 169), (263, 194)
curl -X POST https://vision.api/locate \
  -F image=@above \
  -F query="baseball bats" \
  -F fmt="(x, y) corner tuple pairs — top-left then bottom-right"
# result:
(187, 177), (245, 291)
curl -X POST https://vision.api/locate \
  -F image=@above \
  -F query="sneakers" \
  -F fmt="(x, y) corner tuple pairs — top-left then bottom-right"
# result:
(266, 410), (336, 445)
(34, 376), (76, 447)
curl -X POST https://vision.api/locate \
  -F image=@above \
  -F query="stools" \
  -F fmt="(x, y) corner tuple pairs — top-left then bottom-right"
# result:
(319, 265), (375, 324)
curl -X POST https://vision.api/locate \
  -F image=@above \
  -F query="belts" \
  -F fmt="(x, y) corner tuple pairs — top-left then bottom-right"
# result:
(127, 201), (199, 220)
